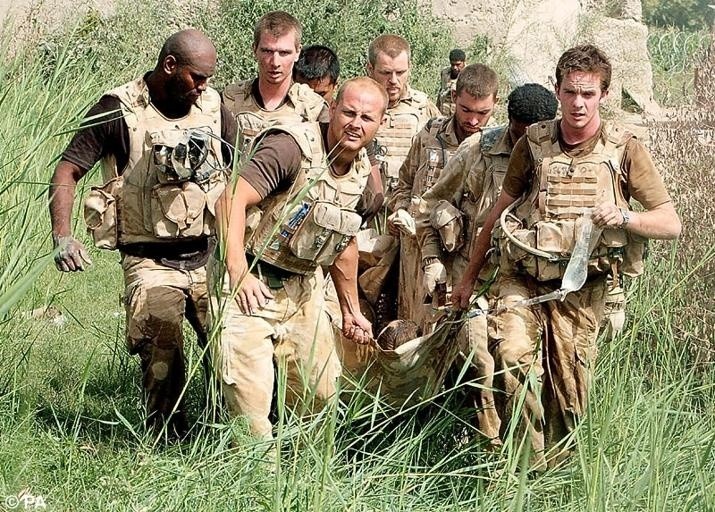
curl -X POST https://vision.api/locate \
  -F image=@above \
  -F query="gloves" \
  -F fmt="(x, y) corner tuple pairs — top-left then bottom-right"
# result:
(423, 258), (447, 303)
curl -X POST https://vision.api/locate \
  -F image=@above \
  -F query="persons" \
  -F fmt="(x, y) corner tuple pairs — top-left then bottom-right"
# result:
(46, 12), (685, 492)
